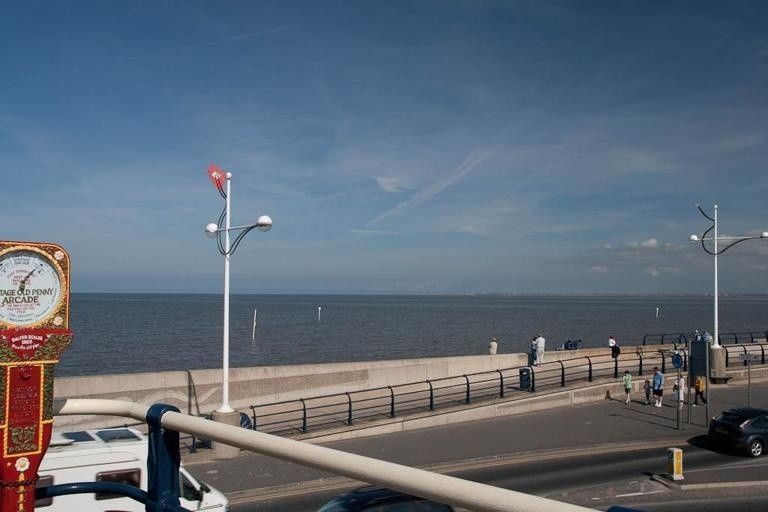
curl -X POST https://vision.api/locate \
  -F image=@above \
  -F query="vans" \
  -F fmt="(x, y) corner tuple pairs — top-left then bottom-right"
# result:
(34, 428), (230, 512)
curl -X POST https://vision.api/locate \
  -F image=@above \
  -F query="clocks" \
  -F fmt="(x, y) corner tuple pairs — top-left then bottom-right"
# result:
(0, 240), (70, 332)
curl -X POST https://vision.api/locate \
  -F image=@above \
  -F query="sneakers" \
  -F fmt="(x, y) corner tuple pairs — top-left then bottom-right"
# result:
(624, 398), (710, 408)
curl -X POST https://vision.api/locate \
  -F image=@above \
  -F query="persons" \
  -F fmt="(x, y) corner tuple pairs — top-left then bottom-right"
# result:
(575, 339), (583, 351)
(530, 336), (538, 366)
(703, 329), (712, 342)
(643, 379), (653, 405)
(652, 366), (665, 407)
(691, 374), (707, 407)
(693, 329), (702, 341)
(487, 337), (498, 355)
(533, 333), (546, 367)
(608, 335), (616, 347)
(623, 369), (633, 405)
(565, 339), (573, 350)
(672, 372), (685, 410)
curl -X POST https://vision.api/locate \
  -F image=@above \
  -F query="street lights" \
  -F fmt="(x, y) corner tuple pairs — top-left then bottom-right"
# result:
(205, 162), (273, 413)
(689, 204), (768, 349)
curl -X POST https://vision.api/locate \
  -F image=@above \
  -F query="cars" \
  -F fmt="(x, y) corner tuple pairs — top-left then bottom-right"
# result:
(317, 485), (455, 512)
(708, 407), (768, 457)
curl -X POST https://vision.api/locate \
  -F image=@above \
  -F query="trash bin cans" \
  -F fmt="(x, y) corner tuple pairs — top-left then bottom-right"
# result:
(519, 368), (530, 391)
